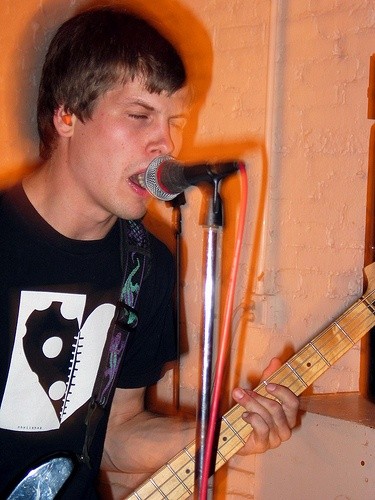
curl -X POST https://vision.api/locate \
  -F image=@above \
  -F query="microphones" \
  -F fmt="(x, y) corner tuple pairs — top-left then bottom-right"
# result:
(143, 154), (240, 201)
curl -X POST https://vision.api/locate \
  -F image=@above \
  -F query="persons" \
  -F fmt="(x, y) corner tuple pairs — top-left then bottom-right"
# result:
(0, 6), (298, 500)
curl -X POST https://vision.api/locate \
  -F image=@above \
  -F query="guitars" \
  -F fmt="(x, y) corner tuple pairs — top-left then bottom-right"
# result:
(6, 262), (375, 500)
